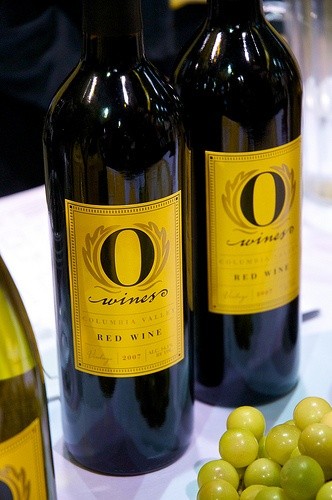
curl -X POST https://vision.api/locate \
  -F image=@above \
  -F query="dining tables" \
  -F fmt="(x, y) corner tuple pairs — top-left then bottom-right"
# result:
(0, 70), (332, 499)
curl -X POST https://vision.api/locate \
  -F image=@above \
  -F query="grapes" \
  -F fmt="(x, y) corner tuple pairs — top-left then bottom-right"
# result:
(197, 398), (331, 500)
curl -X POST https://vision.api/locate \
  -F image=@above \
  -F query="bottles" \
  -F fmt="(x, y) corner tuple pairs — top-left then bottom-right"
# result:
(176, 1), (304, 409)
(41, 0), (195, 478)
(1, 262), (59, 500)
